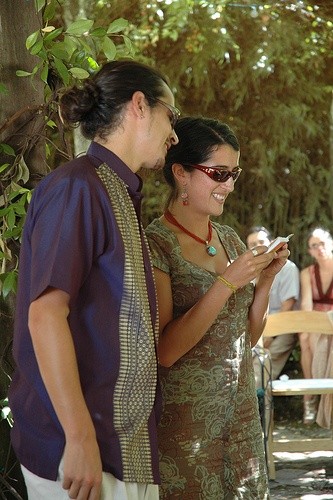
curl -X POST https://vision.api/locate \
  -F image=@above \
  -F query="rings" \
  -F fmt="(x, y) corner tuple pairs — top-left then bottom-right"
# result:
(250, 247), (258, 256)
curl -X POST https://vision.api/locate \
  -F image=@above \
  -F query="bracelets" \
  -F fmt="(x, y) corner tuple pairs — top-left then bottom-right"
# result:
(216, 275), (237, 293)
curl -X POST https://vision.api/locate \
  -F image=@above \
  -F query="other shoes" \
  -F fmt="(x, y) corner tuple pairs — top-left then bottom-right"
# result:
(304, 396), (318, 424)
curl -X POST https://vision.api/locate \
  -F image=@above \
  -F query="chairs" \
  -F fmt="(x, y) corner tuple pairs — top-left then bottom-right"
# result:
(260, 311), (333, 480)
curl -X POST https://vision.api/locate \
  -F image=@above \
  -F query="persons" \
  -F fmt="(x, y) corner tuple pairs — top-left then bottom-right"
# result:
(8, 61), (181, 500)
(244, 225), (333, 430)
(144, 118), (290, 500)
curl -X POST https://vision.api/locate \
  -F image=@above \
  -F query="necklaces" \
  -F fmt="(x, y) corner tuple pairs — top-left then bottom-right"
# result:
(164, 208), (216, 257)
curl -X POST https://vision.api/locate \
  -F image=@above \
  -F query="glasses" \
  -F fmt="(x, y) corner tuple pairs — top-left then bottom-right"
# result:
(309, 241), (325, 249)
(146, 96), (181, 129)
(169, 159), (242, 183)
